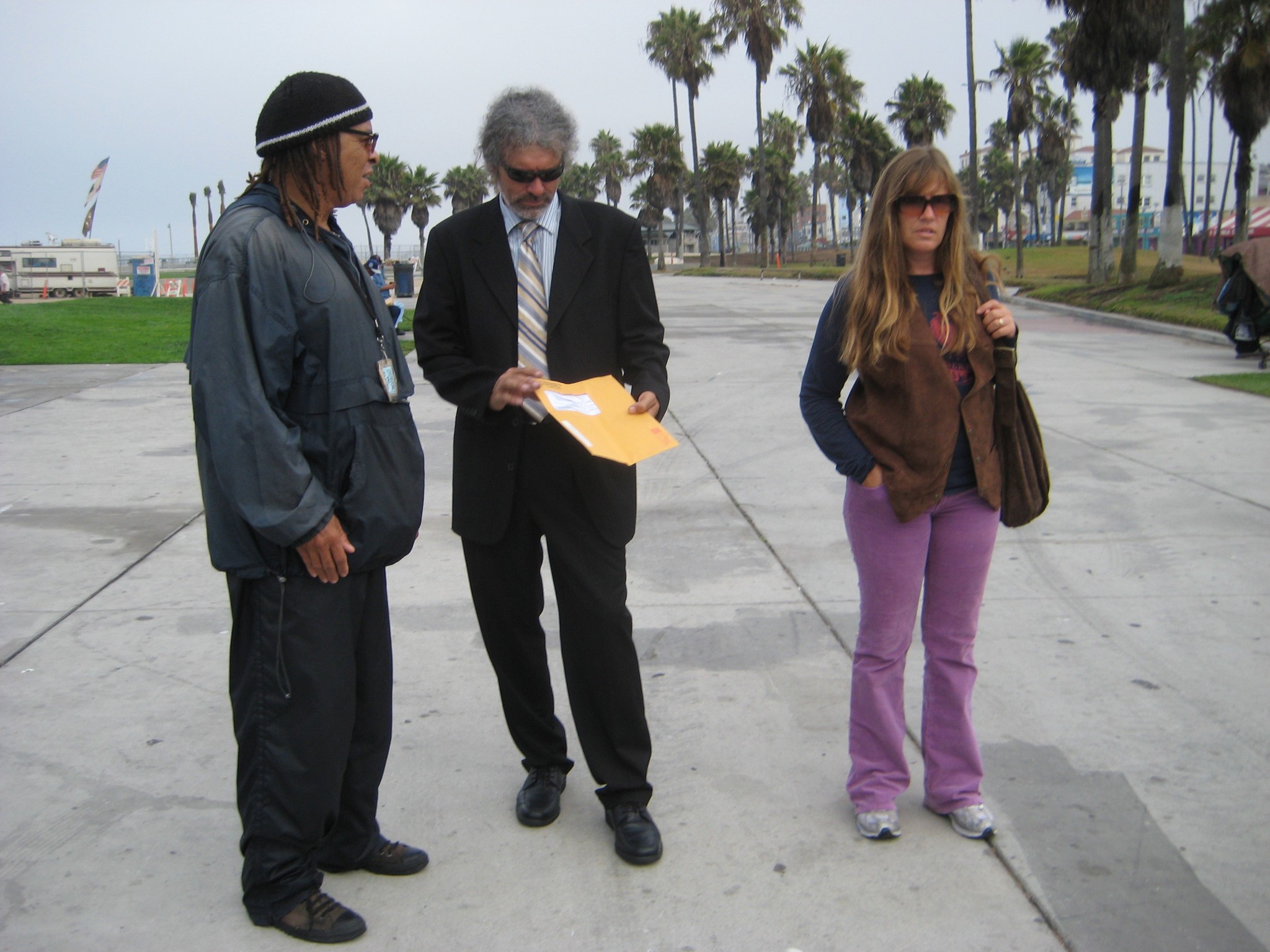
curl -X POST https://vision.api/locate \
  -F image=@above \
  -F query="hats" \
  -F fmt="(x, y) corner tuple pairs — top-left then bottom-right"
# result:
(254, 72), (374, 156)
(367, 259), (381, 274)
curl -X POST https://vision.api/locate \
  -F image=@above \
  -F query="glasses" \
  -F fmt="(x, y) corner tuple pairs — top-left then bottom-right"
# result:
(498, 153), (564, 182)
(893, 192), (958, 217)
(338, 129), (379, 154)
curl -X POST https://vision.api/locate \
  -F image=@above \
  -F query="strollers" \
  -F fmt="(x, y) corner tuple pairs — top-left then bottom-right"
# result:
(1214, 237), (1270, 372)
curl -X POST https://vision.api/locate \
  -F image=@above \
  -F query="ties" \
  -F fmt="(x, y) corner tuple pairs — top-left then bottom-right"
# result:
(517, 222), (551, 422)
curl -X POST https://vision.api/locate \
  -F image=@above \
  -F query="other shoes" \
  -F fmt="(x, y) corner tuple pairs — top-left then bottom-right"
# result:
(395, 328), (405, 335)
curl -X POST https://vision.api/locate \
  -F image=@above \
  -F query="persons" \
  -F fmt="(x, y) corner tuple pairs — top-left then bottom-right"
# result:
(181, 73), (428, 944)
(799, 147), (1019, 840)
(412, 87), (678, 865)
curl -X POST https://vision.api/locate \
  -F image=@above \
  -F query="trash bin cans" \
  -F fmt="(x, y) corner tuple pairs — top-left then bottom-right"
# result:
(835, 254), (847, 266)
(393, 261), (416, 297)
(131, 257), (157, 297)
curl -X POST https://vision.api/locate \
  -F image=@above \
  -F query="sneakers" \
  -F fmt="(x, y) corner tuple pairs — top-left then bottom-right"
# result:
(949, 804), (996, 839)
(272, 890), (367, 942)
(853, 809), (902, 839)
(315, 838), (429, 874)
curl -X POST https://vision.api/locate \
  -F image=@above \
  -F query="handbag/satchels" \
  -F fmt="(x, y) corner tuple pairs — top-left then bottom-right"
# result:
(963, 260), (1049, 529)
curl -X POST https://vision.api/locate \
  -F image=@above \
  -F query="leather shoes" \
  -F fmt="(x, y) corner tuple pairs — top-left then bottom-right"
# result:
(604, 801), (662, 862)
(515, 764), (567, 826)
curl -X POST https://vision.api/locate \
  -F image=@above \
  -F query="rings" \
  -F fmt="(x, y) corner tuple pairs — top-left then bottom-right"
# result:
(999, 318), (1005, 326)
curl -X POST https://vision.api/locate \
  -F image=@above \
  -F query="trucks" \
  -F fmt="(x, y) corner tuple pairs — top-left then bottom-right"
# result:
(0, 237), (119, 298)
